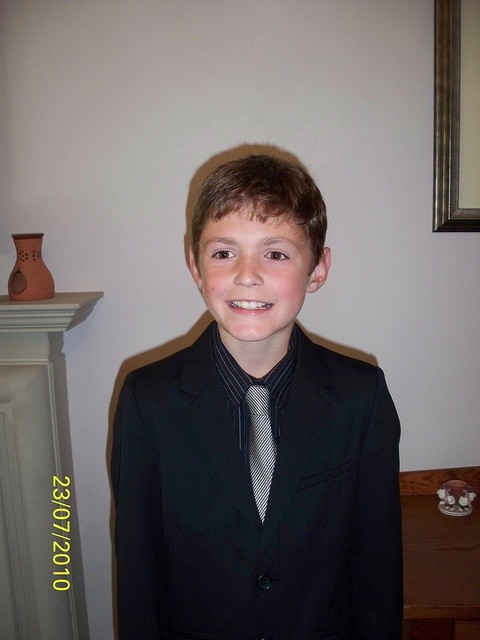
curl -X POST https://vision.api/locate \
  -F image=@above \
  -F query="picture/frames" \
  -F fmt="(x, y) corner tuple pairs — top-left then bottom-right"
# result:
(432, 0), (480, 231)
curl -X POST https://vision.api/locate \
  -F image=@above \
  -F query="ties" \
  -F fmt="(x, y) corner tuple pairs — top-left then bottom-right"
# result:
(245, 385), (278, 522)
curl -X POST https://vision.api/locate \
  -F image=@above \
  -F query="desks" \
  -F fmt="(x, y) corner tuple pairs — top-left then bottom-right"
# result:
(398, 466), (479, 638)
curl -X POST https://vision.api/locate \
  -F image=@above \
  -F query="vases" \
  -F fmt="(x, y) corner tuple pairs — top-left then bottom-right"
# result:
(7, 230), (57, 303)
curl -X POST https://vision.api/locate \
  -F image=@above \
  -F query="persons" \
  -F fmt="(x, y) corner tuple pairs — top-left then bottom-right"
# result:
(107, 154), (401, 639)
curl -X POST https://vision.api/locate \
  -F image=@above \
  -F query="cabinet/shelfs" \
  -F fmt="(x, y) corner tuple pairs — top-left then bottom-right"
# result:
(1, 291), (107, 635)
